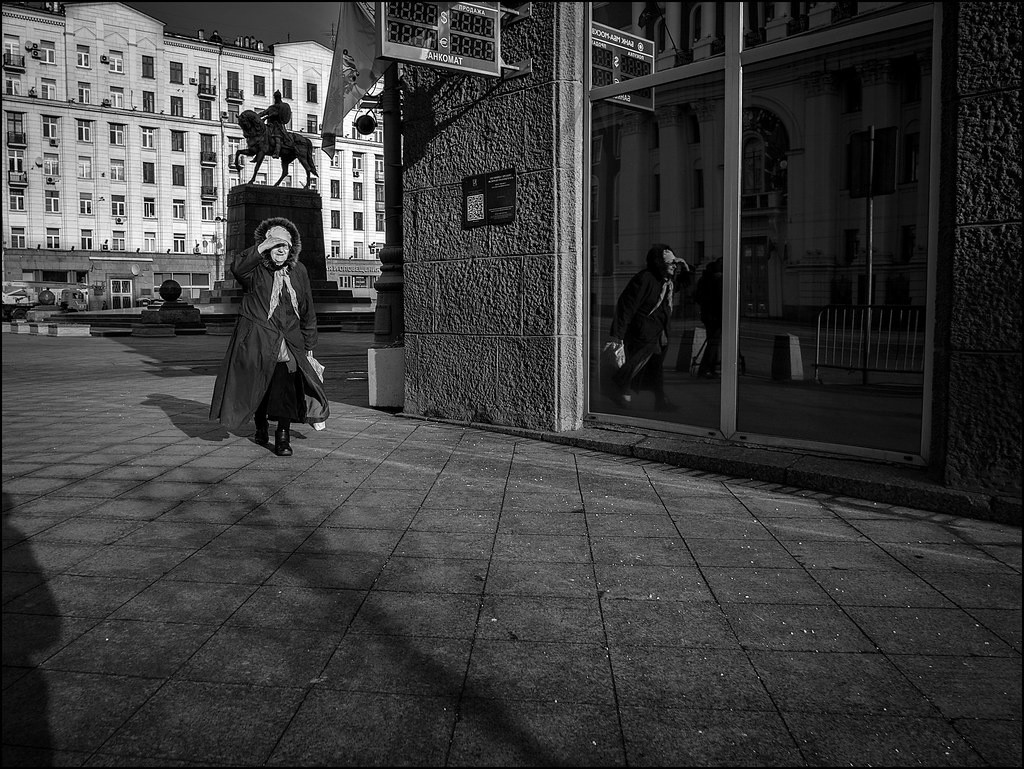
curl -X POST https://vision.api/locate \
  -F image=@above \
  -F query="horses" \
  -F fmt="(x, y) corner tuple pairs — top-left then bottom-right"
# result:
(234, 110), (318, 189)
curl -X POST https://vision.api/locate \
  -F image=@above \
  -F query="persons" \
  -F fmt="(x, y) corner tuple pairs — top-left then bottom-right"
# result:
(608, 242), (694, 411)
(251, 90), (293, 163)
(231, 216), (318, 456)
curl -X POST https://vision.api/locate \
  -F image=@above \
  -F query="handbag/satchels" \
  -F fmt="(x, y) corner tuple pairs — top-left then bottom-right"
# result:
(306, 350), (326, 431)
(601, 340), (632, 402)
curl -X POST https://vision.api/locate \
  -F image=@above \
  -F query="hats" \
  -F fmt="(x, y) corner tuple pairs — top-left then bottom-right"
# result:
(265, 226), (292, 247)
(663, 249), (676, 260)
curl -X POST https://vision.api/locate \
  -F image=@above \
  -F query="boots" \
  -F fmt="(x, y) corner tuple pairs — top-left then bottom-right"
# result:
(656, 393), (676, 410)
(613, 396), (633, 409)
(254, 418), (270, 444)
(275, 430), (293, 456)
